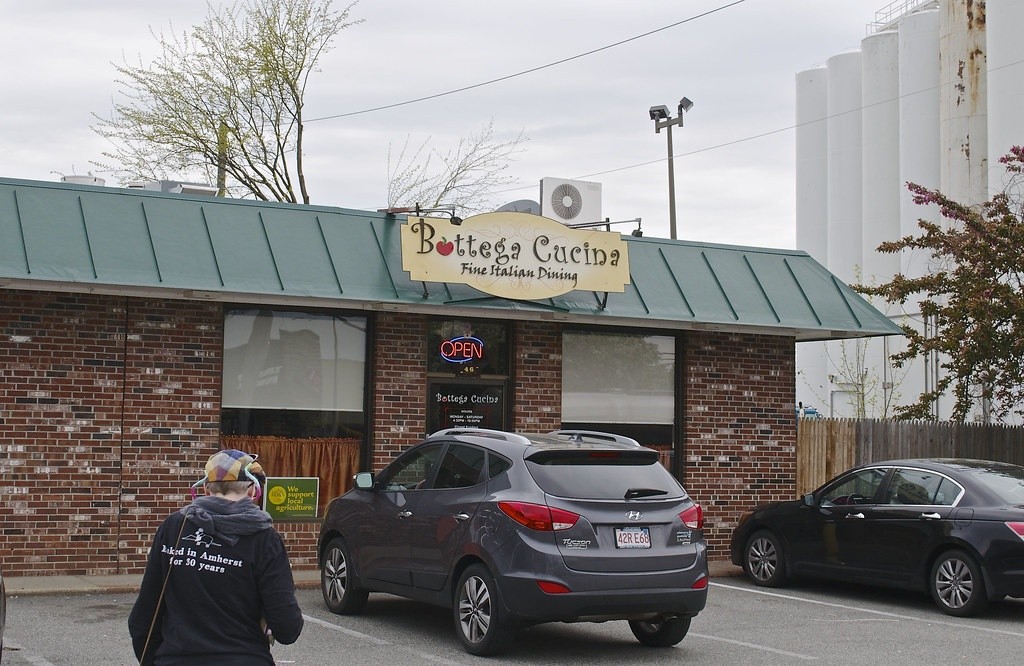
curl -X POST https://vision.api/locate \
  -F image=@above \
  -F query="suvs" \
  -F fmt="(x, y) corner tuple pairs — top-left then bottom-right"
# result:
(317, 428), (710, 655)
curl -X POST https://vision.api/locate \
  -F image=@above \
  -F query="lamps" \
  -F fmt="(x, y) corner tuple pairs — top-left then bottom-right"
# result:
(377, 202), (463, 226)
(571, 217), (643, 238)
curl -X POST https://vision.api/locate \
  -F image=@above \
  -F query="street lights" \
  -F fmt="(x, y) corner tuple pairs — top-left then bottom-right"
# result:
(649, 96), (694, 239)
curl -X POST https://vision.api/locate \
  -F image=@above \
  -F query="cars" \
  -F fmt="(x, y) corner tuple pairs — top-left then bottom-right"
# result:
(731, 457), (1024, 618)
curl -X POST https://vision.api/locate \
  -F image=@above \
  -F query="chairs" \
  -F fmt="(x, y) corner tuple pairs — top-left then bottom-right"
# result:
(896, 483), (930, 505)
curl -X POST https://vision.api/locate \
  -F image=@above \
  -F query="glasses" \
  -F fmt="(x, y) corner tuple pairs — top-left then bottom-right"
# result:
(245, 454), (258, 467)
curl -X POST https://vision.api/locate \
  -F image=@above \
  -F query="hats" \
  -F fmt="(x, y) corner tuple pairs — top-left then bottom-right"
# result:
(205, 449), (266, 484)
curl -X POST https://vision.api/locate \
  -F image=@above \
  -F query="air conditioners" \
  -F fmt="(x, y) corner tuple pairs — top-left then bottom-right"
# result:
(538, 176), (603, 231)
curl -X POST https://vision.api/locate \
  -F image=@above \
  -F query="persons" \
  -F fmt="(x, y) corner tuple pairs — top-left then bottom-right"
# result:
(128, 449), (304, 666)
